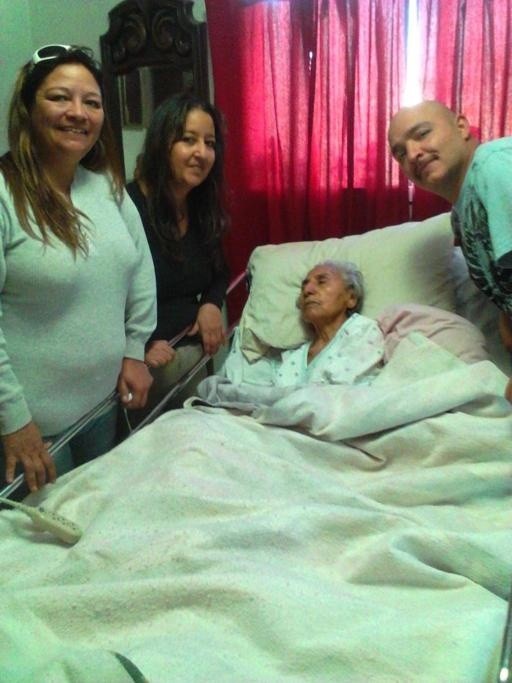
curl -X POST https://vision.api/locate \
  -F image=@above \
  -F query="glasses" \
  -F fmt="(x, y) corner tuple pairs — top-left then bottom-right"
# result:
(29, 44), (103, 88)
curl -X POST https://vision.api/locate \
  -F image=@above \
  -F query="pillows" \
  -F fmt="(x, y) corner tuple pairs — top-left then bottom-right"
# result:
(239, 212), (494, 371)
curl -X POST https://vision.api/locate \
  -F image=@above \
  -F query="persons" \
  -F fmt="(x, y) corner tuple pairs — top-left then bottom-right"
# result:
(0, 41), (159, 503)
(267, 258), (387, 392)
(119, 92), (228, 440)
(386, 100), (512, 408)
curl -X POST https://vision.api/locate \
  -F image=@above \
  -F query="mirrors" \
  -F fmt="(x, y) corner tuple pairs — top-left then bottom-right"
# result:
(112, 64), (198, 189)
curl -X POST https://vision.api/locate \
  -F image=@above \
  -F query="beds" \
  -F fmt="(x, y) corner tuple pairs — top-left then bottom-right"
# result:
(0, 210), (511, 683)
